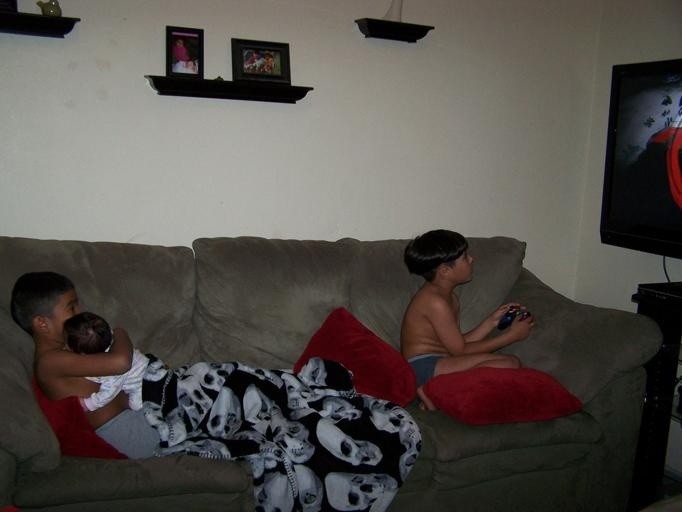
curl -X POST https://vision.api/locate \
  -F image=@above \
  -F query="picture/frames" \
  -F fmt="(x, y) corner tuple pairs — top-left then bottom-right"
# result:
(166, 26), (204, 79)
(231, 37), (292, 86)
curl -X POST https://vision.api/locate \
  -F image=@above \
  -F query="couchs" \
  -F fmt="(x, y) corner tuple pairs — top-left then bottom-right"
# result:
(0, 234), (663, 512)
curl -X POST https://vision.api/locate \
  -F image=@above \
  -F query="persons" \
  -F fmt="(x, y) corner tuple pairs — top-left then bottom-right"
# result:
(173, 39), (196, 73)
(401, 230), (535, 411)
(244, 51), (274, 73)
(64, 312), (150, 412)
(10, 271), (133, 430)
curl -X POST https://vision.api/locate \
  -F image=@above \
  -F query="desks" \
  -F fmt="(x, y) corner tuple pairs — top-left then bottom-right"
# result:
(632, 282), (682, 501)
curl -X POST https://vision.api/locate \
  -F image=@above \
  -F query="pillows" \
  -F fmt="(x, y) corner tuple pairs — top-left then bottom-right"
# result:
(423, 367), (583, 425)
(293, 306), (418, 409)
(356, 239), (525, 355)
(2, 242), (193, 472)
(194, 241), (354, 365)
